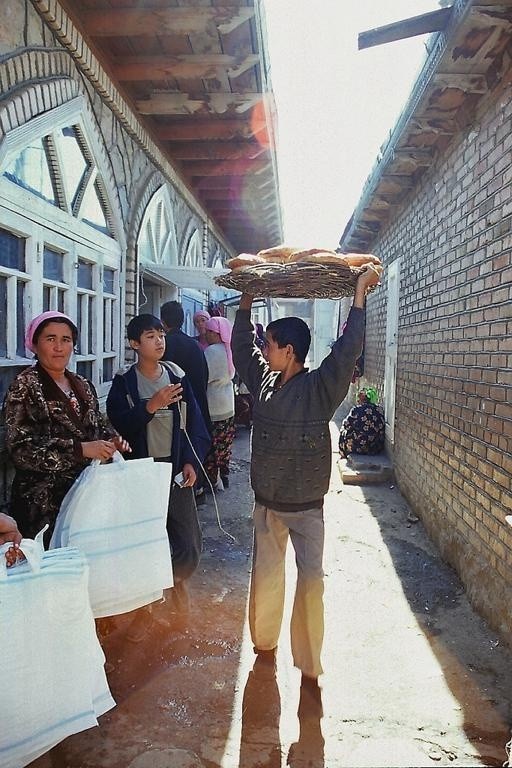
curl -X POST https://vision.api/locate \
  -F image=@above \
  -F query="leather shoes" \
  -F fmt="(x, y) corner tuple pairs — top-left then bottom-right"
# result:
(124, 612), (154, 644)
(171, 581), (189, 616)
(253, 649), (276, 682)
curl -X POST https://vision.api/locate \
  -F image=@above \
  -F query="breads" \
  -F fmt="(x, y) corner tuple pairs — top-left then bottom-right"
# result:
(227, 246), (380, 272)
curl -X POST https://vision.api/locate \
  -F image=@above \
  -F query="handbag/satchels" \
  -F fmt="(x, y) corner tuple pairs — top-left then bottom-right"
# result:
(50, 449), (175, 618)
(0, 540), (118, 768)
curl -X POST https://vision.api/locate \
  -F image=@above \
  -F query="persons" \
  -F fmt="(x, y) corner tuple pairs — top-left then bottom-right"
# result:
(105, 313), (210, 641)
(160, 299), (273, 492)
(0, 513), (25, 567)
(230, 261), (381, 674)
(338, 386), (386, 460)
(5, 310), (131, 673)
